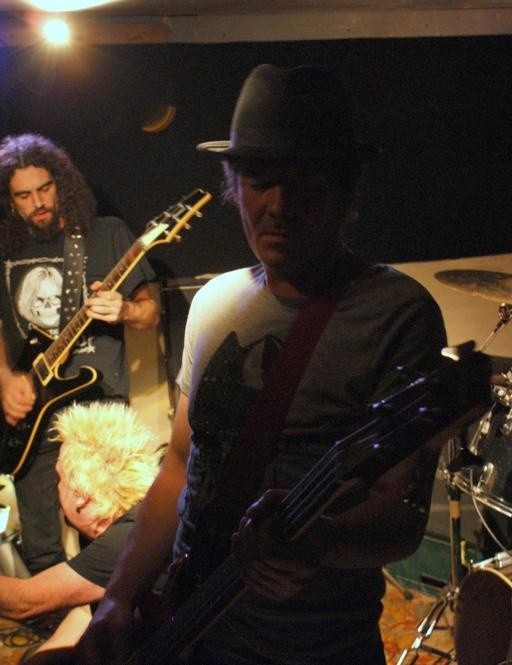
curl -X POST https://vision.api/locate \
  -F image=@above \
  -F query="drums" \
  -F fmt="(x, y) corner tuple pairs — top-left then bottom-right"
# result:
(453, 550), (512, 664)
(453, 383), (512, 519)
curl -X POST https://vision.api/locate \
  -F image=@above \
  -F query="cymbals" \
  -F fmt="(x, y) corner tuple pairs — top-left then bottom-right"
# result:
(434, 269), (512, 305)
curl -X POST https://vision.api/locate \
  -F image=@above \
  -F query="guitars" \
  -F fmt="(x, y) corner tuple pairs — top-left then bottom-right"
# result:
(0, 189), (212, 475)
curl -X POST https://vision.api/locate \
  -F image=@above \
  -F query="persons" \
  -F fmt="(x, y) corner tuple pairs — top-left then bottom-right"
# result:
(0, 131), (164, 577)
(0, 400), (163, 652)
(73, 63), (449, 665)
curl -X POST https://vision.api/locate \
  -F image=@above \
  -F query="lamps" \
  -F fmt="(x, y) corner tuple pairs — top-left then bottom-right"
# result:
(127, 91), (177, 140)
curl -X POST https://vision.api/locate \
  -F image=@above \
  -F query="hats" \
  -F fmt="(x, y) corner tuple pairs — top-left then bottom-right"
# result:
(197, 62), (387, 168)
